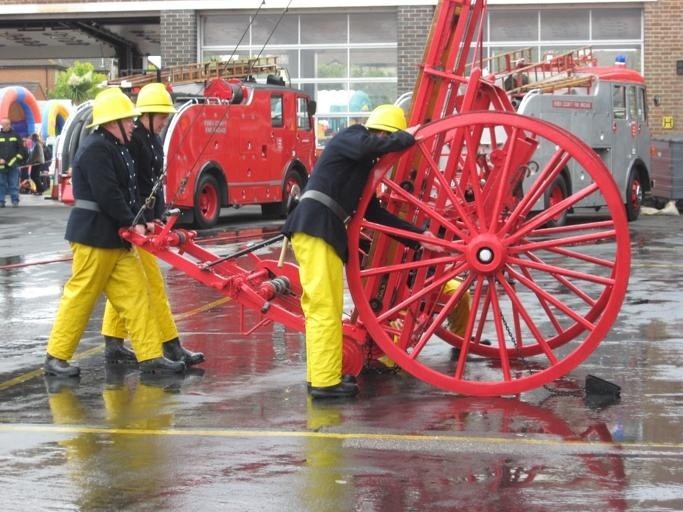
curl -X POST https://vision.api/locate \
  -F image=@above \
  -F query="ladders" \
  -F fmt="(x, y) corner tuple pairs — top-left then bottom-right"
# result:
(452, 44), (594, 97)
(164, 56), (279, 86)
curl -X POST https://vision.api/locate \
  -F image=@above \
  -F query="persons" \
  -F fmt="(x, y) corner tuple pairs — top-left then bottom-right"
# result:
(280, 104), (445, 400)
(43, 87), (187, 379)
(26, 133), (45, 195)
(345, 217), (491, 368)
(0, 116), (25, 209)
(99, 80), (206, 366)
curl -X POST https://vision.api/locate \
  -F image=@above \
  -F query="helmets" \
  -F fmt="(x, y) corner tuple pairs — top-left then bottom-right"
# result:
(85, 88), (143, 128)
(363, 103), (408, 132)
(135, 82), (179, 114)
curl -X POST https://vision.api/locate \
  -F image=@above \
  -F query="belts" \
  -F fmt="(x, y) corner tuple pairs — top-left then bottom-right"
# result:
(300, 190), (352, 229)
(140, 193), (158, 211)
(74, 198), (101, 213)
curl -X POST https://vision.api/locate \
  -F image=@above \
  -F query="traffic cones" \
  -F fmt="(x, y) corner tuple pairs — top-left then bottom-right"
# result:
(44, 150), (58, 201)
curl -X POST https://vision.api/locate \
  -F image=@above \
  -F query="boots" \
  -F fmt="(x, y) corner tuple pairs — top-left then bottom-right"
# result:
(139, 356), (186, 374)
(42, 353), (80, 376)
(162, 337), (205, 365)
(104, 335), (138, 362)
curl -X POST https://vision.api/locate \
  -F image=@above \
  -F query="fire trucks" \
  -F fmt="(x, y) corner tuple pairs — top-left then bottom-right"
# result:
(59, 59), (317, 227)
(390, 45), (659, 227)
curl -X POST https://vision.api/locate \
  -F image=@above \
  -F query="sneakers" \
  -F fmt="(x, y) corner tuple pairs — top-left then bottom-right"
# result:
(452, 340), (491, 361)
(307, 374), (360, 396)
